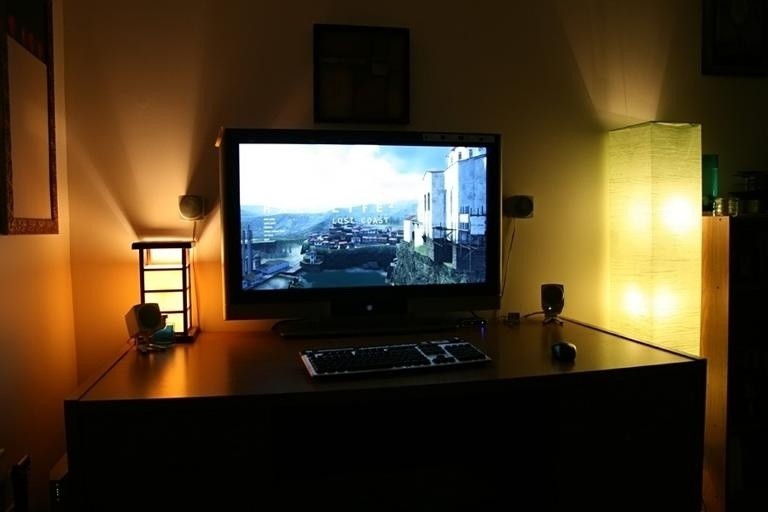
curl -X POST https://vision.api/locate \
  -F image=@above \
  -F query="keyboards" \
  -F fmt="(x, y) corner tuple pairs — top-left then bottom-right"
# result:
(299, 339), (494, 382)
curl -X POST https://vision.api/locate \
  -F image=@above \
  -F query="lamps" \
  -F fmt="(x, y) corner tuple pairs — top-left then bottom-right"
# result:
(134, 242), (199, 343)
(606, 119), (702, 358)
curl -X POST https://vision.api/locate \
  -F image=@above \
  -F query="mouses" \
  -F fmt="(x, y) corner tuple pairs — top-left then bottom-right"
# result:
(551, 340), (579, 365)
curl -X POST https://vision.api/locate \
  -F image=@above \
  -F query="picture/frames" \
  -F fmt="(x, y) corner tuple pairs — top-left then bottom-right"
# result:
(699, 0), (768, 78)
(1, 1), (60, 235)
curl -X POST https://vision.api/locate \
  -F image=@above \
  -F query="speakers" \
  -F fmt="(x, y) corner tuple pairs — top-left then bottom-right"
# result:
(541, 282), (566, 324)
(503, 194), (536, 221)
(123, 303), (166, 356)
(177, 193), (210, 220)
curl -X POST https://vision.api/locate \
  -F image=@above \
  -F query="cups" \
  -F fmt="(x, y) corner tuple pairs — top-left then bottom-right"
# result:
(713, 197), (739, 219)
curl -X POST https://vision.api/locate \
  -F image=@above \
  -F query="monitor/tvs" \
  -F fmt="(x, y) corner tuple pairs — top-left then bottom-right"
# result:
(217, 129), (501, 336)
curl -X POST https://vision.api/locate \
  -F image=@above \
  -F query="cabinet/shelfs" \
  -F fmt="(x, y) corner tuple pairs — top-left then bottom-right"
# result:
(702, 209), (768, 512)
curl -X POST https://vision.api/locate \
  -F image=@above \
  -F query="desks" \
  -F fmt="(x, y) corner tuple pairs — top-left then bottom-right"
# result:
(62, 316), (708, 512)
